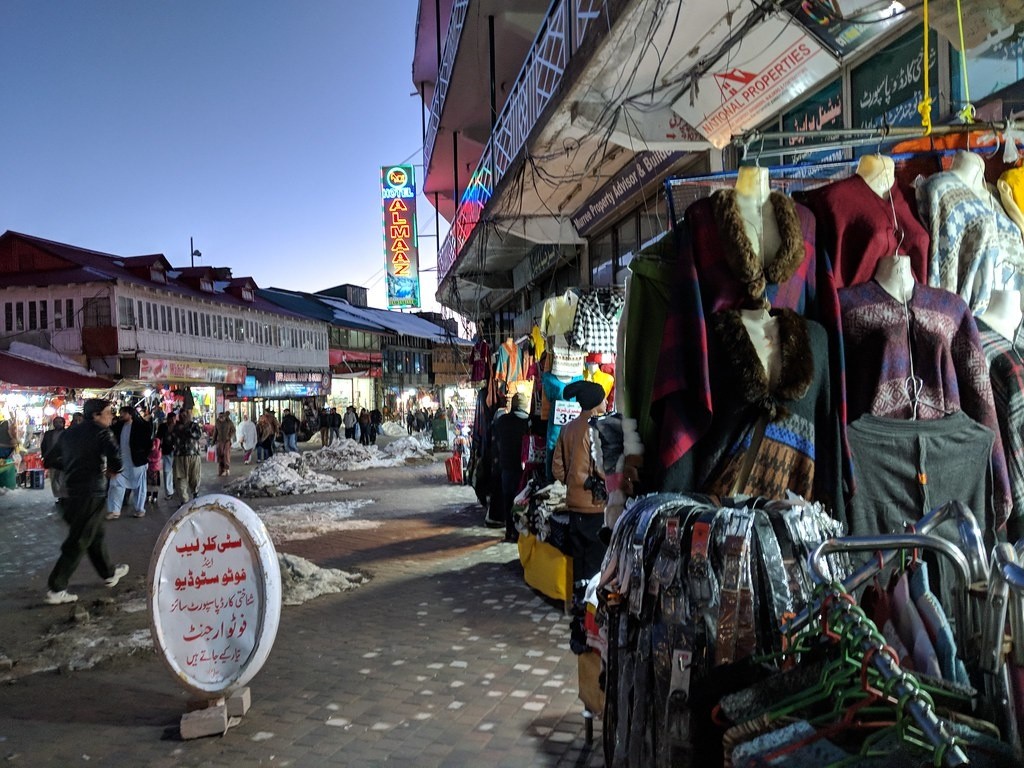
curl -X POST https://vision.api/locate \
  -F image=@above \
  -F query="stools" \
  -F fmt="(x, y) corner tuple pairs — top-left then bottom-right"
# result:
(25, 468), (44, 489)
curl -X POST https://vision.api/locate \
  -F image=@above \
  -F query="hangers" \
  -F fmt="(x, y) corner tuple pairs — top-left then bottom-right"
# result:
(712, 521), (975, 767)
(722, 114), (1023, 420)
(470, 282), (621, 423)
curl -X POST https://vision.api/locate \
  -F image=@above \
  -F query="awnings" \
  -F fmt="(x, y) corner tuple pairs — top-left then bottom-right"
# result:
(0, 341), (117, 390)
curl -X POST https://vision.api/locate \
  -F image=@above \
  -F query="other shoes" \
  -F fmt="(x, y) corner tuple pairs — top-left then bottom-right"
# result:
(134, 512), (145, 518)
(165, 495), (172, 500)
(105, 513), (121, 520)
(146, 497), (156, 503)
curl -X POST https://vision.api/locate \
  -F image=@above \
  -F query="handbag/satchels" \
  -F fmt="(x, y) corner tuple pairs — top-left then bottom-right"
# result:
(377, 424), (383, 435)
(445, 453), (462, 485)
(206, 445), (218, 462)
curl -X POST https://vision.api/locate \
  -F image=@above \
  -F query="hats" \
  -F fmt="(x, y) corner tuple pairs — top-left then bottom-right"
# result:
(512, 393), (528, 409)
(564, 381), (605, 411)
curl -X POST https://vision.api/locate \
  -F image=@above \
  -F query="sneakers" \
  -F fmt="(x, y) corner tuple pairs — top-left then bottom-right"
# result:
(43, 590), (79, 604)
(104, 564), (129, 588)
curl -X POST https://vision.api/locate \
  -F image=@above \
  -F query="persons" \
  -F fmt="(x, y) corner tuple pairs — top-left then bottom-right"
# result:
(500, 141), (1024, 504)
(42, 398), (129, 605)
(490, 393), (531, 543)
(0, 392), (462, 521)
(549, 378), (609, 615)
